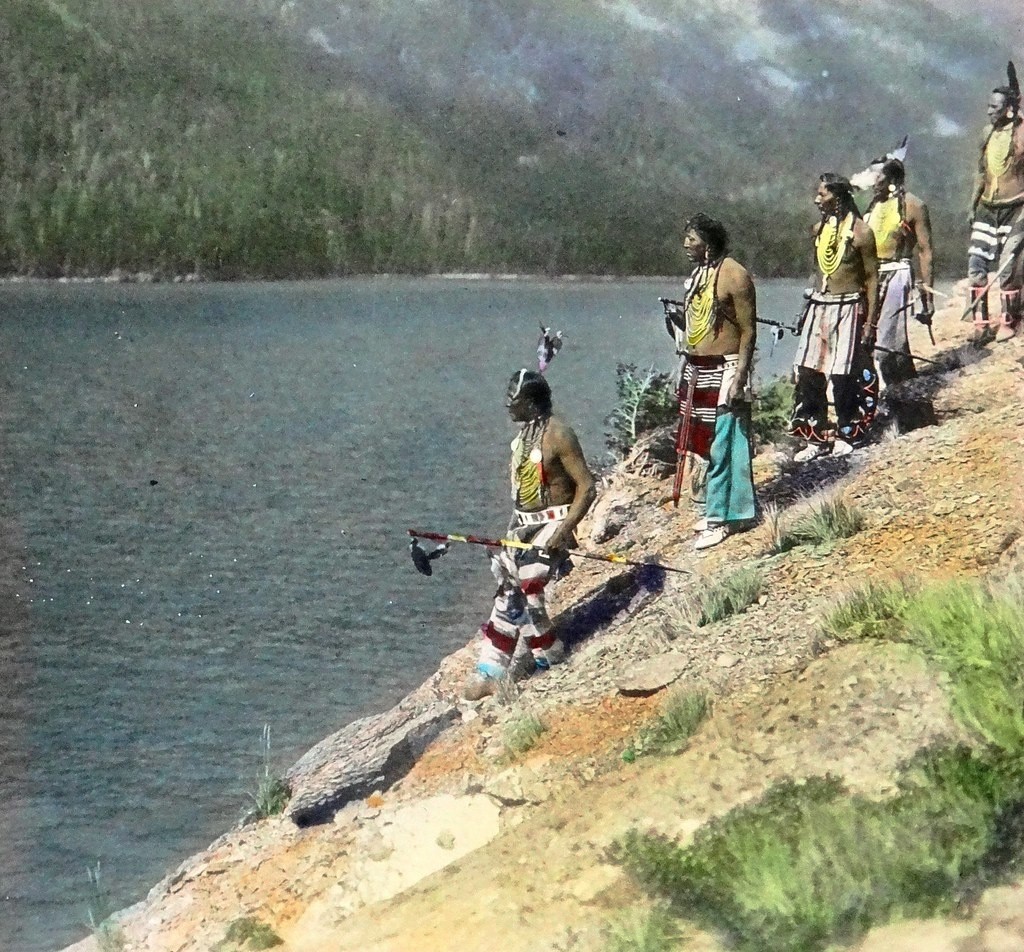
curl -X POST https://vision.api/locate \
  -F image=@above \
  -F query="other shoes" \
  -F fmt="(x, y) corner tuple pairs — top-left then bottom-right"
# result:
(466, 679), (496, 701)
(794, 444), (832, 462)
(521, 663), (550, 680)
(694, 518), (745, 548)
(832, 440), (854, 458)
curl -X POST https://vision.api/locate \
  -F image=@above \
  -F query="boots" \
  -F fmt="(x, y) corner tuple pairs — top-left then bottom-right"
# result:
(996, 288), (1020, 341)
(967, 287), (991, 342)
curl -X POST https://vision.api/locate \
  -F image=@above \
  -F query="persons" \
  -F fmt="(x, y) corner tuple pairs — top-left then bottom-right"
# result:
(965, 86), (1024, 342)
(792, 172), (878, 463)
(863, 156), (935, 417)
(676, 211), (757, 548)
(477, 369), (597, 678)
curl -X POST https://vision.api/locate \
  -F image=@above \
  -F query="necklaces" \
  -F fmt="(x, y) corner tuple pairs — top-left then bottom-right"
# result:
(867, 198), (901, 246)
(519, 413), (549, 506)
(685, 259), (722, 346)
(985, 123), (1014, 176)
(816, 211), (854, 274)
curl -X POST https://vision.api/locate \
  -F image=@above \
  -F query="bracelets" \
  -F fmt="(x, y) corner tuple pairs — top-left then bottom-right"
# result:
(862, 322), (878, 329)
